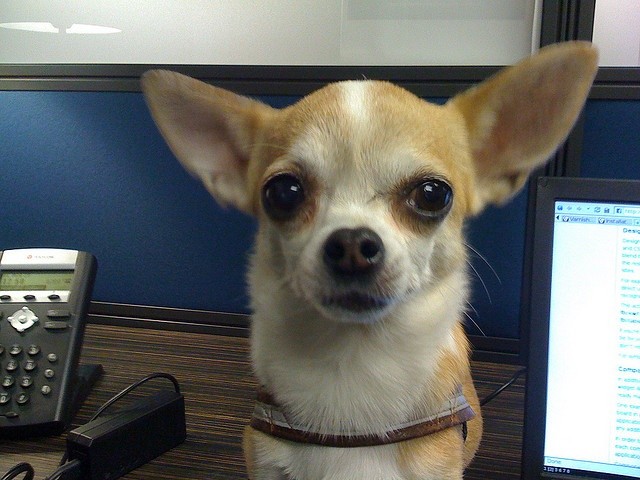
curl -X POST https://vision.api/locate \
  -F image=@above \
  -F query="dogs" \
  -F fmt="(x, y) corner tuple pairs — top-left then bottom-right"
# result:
(139, 38), (602, 480)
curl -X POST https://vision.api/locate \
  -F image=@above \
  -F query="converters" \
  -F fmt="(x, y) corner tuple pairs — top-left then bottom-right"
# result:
(63, 387), (190, 479)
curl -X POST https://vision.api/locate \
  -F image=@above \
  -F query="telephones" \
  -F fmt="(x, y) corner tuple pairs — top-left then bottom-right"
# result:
(0, 246), (104, 439)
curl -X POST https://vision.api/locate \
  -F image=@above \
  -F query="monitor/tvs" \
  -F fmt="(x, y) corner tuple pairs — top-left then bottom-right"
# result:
(520, 173), (640, 480)
